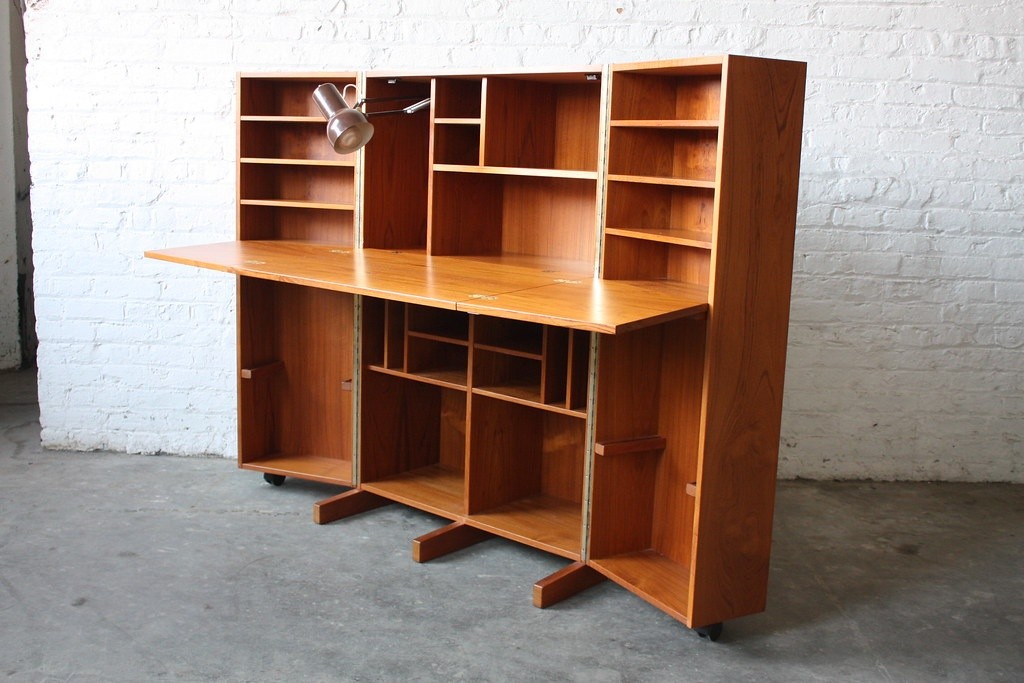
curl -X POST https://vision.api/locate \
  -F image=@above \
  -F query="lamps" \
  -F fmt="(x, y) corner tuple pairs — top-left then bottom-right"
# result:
(310, 82), (433, 154)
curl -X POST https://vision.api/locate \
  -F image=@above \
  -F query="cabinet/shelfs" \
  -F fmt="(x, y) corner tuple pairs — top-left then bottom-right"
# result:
(144, 56), (808, 631)
